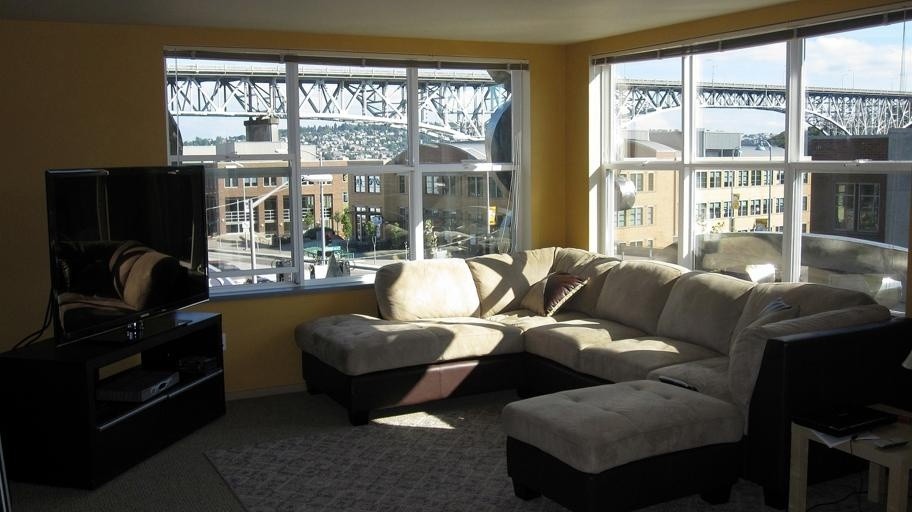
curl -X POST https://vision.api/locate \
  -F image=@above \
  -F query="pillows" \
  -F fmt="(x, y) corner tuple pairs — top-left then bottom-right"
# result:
(519, 273), (590, 319)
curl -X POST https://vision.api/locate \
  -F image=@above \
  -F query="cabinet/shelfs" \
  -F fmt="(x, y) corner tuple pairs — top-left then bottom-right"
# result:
(2, 313), (228, 493)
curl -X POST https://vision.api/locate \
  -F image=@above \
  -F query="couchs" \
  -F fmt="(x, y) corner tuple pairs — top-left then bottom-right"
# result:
(291, 244), (912, 511)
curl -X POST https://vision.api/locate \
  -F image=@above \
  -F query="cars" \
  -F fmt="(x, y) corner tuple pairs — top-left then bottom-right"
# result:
(207, 260), (253, 287)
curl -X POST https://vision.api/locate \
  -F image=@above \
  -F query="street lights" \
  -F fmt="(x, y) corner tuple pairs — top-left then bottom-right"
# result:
(249, 173), (333, 284)
(275, 149), (326, 265)
(756, 139), (772, 232)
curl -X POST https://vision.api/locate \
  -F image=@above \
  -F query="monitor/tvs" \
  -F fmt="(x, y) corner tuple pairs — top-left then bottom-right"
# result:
(44, 164), (211, 349)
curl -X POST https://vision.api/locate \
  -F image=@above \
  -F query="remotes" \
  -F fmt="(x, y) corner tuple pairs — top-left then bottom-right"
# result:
(659, 374), (700, 393)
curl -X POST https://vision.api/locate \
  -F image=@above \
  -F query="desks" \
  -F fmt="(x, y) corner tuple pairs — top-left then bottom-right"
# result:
(786, 404), (912, 512)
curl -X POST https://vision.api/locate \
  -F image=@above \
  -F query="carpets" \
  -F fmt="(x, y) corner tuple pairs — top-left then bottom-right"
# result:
(205, 395), (790, 512)
